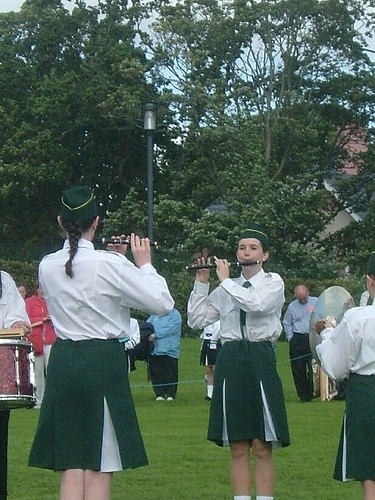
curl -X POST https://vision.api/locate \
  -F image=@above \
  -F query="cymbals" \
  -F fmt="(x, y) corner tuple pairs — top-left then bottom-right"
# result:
(308, 285), (356, 367)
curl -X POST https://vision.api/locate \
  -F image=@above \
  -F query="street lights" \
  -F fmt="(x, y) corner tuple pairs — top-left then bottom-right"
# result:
(139, 91), (160, 245)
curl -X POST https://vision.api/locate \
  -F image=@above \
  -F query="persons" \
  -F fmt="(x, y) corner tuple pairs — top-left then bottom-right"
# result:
(28, 186), (175, 500)
(126, 318), (141, 373)
(187, 225), (286, 500)
(0, 270), (56, 500)
(283, 285), (319, 403)
(146, 309), (182, 402)
(200, 320), (220, 400)
(314, 255), (375, 500)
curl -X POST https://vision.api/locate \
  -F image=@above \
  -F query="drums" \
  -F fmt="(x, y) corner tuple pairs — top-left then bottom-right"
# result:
(0, 338), (37, 410)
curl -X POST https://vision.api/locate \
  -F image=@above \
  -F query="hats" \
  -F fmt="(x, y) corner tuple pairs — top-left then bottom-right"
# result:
(240, 223), (270, 248)
(367, 251), (375, 280)
(60, 185), (98, 221)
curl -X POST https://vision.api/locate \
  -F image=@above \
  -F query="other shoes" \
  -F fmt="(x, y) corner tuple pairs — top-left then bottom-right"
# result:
(204, 396), (211, 401)
(156, 397), (164, 400)
(166, 397), (173, 400)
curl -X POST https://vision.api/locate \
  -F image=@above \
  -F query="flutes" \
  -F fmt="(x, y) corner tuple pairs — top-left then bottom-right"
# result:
(185, 260), (260, 270)
(91, 236), (158, 247)
